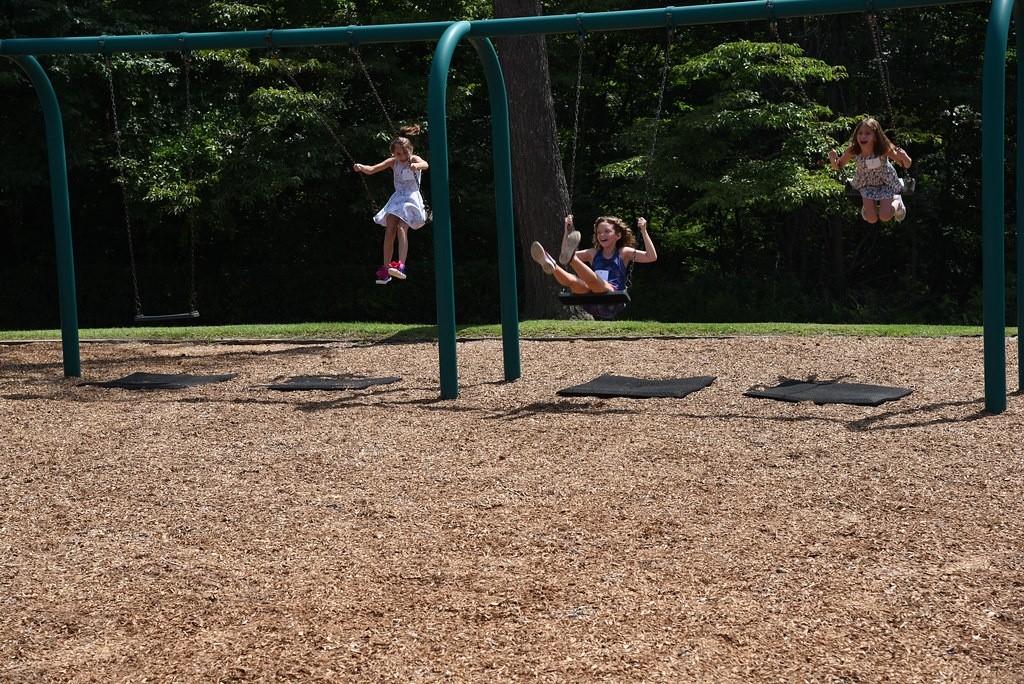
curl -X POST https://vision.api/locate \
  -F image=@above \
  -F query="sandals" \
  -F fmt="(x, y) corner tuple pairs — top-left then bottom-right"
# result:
(894, 195), (906, 221)
(861, 205), (867, 220)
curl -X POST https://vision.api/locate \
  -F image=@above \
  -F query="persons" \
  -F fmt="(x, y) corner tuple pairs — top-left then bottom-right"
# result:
(353, 137), (428, 284)
(829, 118), (912, 223)
(531, 215), (657, 318)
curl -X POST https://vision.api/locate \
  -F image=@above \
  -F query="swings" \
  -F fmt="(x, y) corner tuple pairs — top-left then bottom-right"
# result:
(556, 28), (674, 305)
(103, 52), (202, 323)
(268, 46), (434, 224)
(768, 14), (916, 198)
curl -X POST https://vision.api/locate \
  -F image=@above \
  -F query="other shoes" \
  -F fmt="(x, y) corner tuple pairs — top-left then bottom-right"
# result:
(375, 265), (392, 284)
(530, 241), (556, 274)
(388, 261), (407, 279)
(558, 230), (581, 265)
